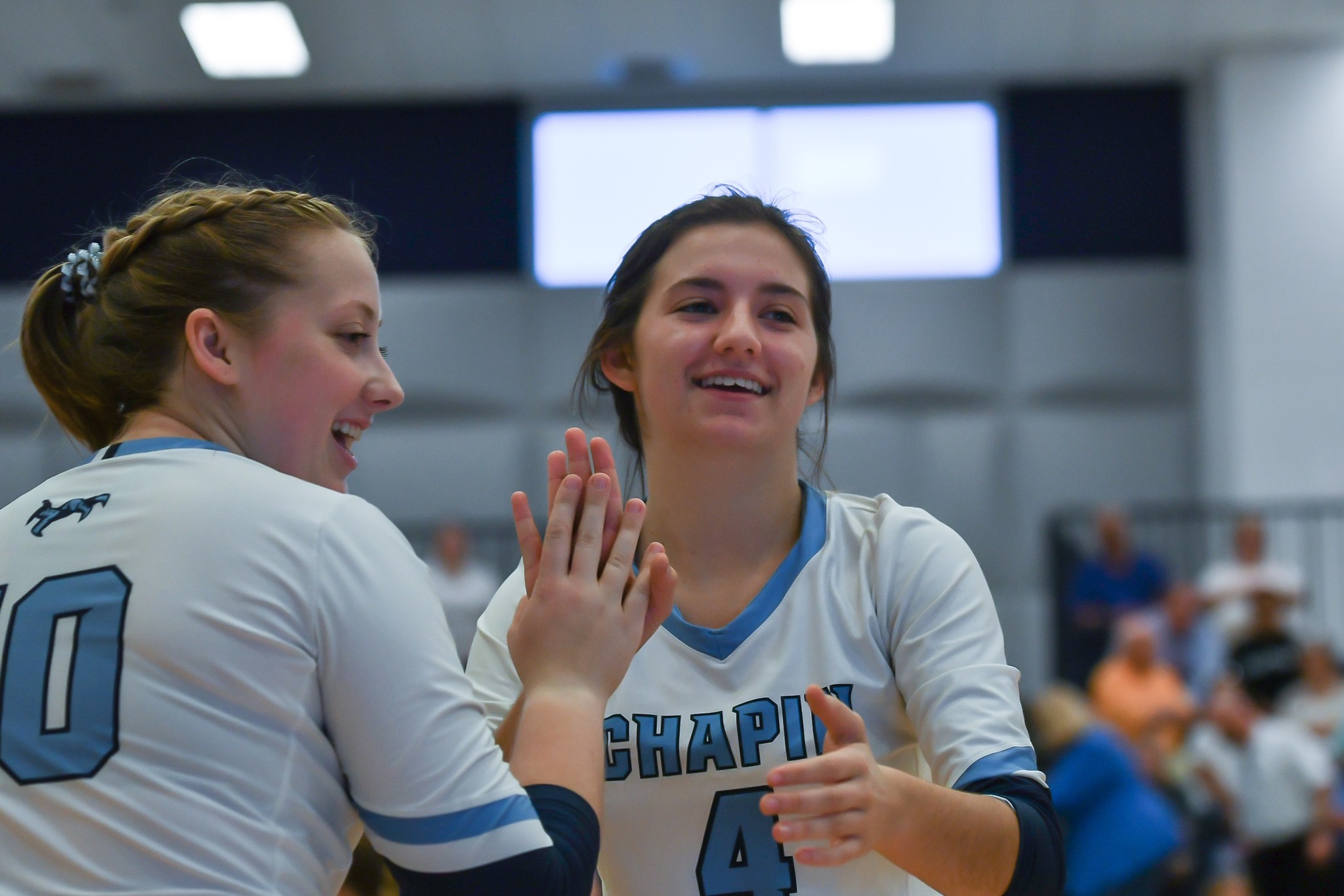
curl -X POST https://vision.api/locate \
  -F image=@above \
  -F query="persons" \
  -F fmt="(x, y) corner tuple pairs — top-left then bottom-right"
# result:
(426, 182), (1344, 896)
(2, 182), (666, 896)
